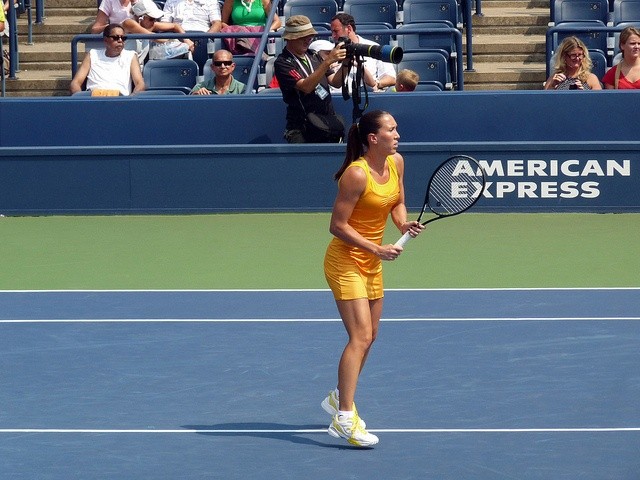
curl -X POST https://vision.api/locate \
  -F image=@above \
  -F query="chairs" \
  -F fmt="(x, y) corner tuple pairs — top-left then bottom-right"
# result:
(356, 22), (394, 46)
(550, 0), (612, 21)
(203, 56), (261, 89)
(403, 0), (458, 27)
(142, 60), (199, 85)
(553, 21), (611, 49)
(614, 22), (639, 53)
(283, 0), (339, 22)
(398, 49), (450, 86)
(414, 80), (443, 93)
(343, 0), (398, 22)
(398, 20), (456, 55)
(553, 52), (610, 79)
(614, 1), (639, 21)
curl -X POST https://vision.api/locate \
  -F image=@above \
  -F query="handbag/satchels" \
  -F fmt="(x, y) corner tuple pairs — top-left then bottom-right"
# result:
(297, 91), (345, 143)
(555, 77), (592, 90)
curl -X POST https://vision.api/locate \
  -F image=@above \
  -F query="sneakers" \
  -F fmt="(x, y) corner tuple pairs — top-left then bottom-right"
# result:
(328, 415), (379, 446)
(321, 391), (366, 428)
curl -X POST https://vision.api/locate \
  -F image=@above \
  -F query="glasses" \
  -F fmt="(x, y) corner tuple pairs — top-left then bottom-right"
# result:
(109, 35), (127, 41)
(147, 17), (155, 21)
(565, 52), (585, 60)
(214, 61), (233, 66)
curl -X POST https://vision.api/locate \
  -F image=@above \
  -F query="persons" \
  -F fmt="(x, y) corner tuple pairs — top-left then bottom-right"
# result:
(320, 109), (427, 448)
(222, 0), (282, 57)
(601, 27), (640, 90)
(90, 0), (136, 34)
(395, 69), (419, 92)
(331, 14), (396, 94)
(0, 0), (10, 73)
(187, 49), (247, 95)
(274, 15), (351, 144)
(119, 0), (194, 74)
(307, 40), (339, 94)
(544, 36), (603, 90)
(69, 23), (147, 98)
(160, 0), (222, 76)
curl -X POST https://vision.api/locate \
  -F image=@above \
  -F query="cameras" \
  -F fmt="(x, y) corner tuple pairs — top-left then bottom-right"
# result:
(335, 36), (404, 65)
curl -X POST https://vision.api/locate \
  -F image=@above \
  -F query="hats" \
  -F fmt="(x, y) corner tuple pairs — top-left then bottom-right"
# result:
(308, 40), (334, 50)
(282, 15), (318, 40)
(132, 0), (164, 19)
